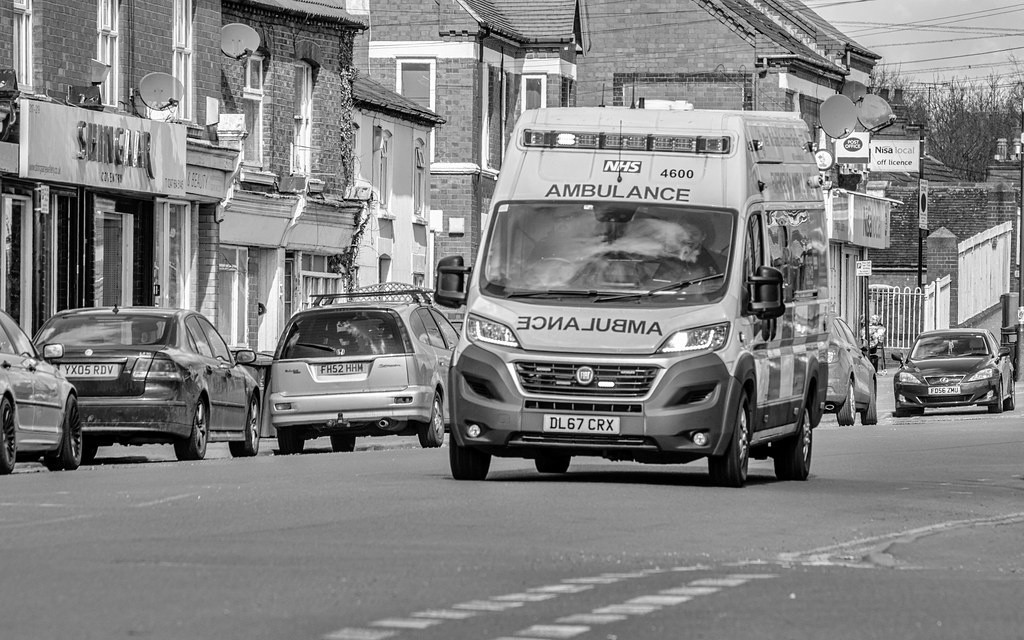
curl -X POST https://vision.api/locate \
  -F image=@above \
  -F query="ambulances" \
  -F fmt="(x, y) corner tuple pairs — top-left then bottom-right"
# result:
(434, 97), (833, 488)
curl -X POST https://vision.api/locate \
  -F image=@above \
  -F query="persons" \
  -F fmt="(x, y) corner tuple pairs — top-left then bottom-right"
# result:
(526, 203), (592, 277)
(652, 213), (722, 286)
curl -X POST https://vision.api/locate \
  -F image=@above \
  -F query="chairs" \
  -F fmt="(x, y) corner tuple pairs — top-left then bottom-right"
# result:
(964, 338), (986, 354)
(650, 242), (720, 283)
(331, 331), (358, 355)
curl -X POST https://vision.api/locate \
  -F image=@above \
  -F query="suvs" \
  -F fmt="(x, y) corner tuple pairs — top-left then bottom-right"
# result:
(268, 288), (460, 454)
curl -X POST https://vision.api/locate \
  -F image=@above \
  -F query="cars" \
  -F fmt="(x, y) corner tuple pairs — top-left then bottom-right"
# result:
(30, 306), (262, 460)
(0, 307), (82, 474)
(824, 311), (878, 426)
(889, 327), (1015, 418)
